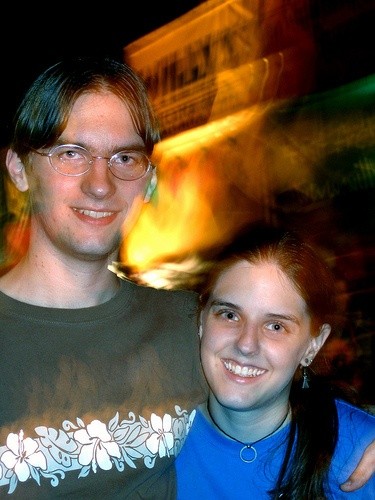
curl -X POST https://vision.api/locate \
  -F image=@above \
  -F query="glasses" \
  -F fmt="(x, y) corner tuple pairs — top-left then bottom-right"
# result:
(21, 144), (154, 182)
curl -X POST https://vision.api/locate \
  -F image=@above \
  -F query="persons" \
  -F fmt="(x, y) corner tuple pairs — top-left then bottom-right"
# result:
(0, 56), (375, 500)
(175, 236), (375, 500)
